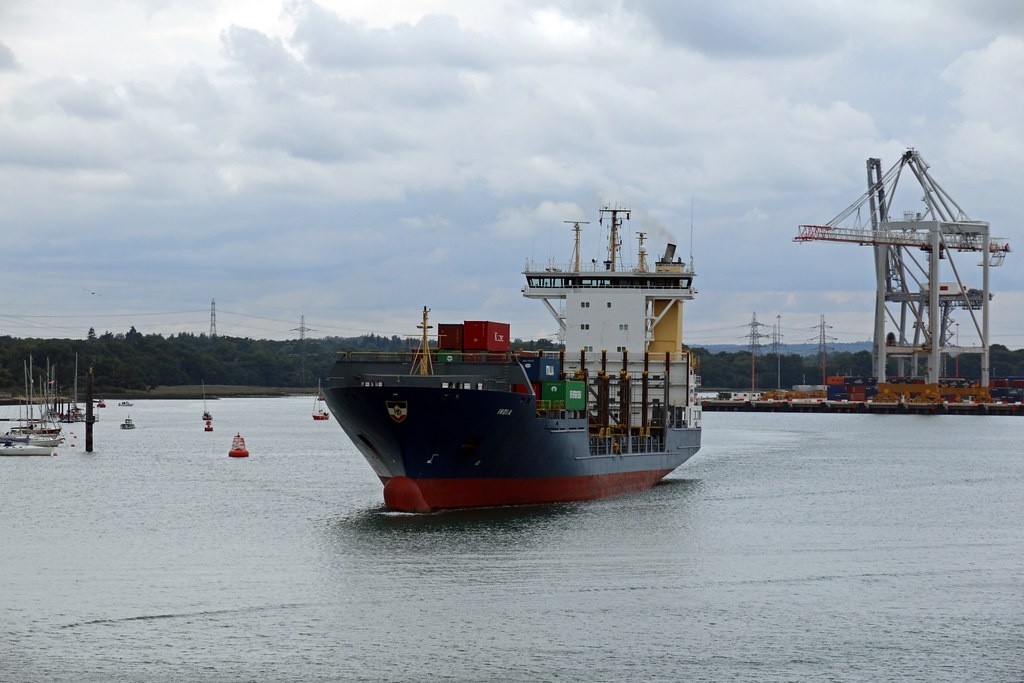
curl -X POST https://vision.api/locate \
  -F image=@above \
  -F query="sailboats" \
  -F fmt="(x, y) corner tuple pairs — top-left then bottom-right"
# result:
(0, 360), (53, 456)
(0, 355), (65, 447)
(42, 351), (99, 423)
(10, 375), (62, 434)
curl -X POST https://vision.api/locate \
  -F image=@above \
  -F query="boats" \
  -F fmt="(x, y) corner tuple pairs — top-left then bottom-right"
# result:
(320, 207), (702, 514)
(312, 409), (329, 420)
(204, 420), (213, 431)
(96, 398), (106, 408)
(119, 414), (135, 429)
(118, 401), (136, 406)
(228, 432), (249, 457)
(317, 392), (325, 401)
(202, 411), (212, 420)
